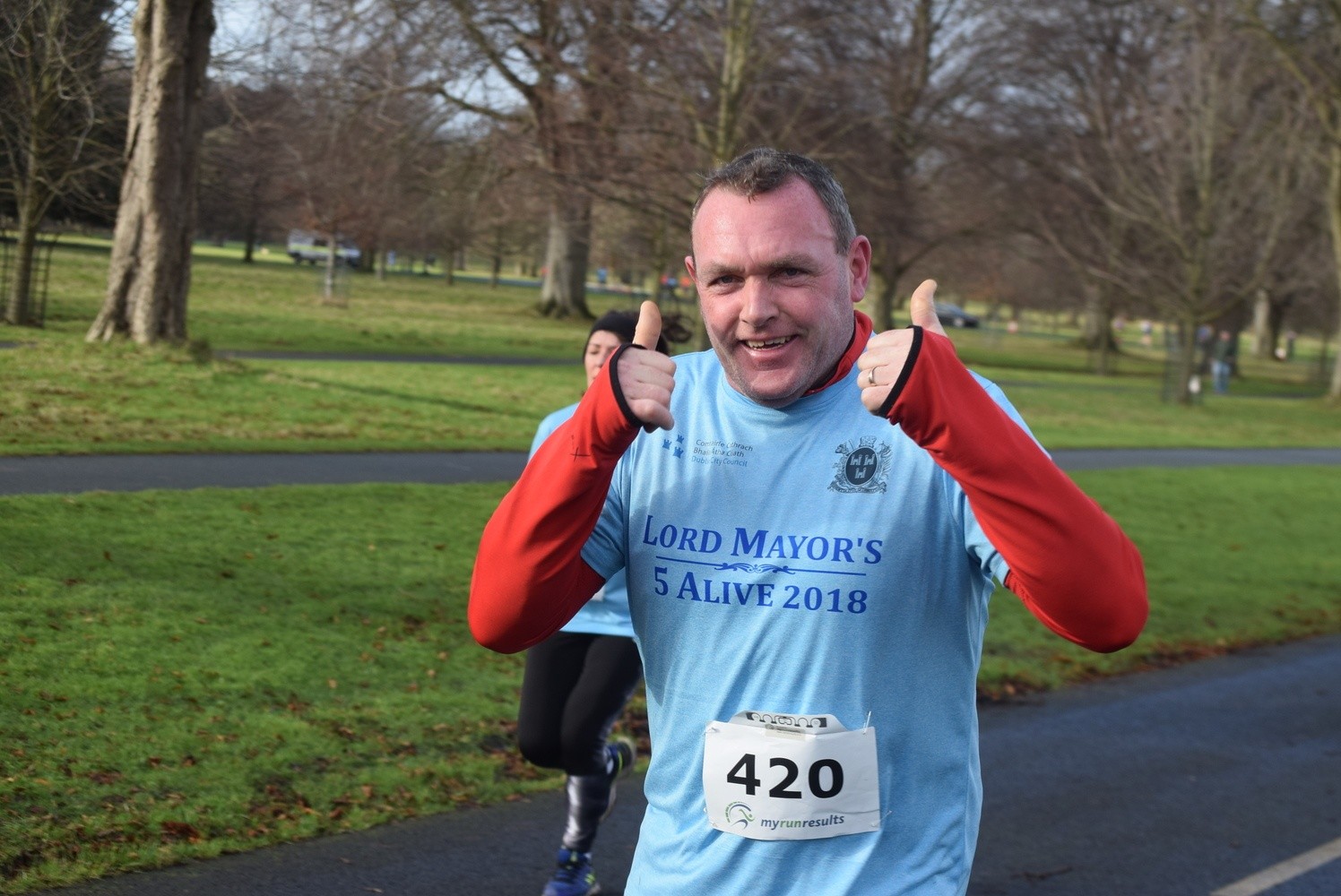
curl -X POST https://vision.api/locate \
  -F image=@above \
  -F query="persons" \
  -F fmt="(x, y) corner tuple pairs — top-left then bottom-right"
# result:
(466, 150), (1148, 896)
(1211, 329), (1233, 393)
(513, 311), (692, 896)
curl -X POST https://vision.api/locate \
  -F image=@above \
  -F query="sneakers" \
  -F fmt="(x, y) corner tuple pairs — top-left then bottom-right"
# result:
(598, 738), (635, 822)
(543, 846), (601, 896)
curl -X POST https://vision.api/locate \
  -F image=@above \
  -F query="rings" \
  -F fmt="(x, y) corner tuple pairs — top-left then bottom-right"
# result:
(868, 366), (878, 387)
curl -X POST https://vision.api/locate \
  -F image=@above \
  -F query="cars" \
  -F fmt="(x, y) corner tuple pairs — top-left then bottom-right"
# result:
(934, 302), (980, 329)
(286, 227), (363, 268)
